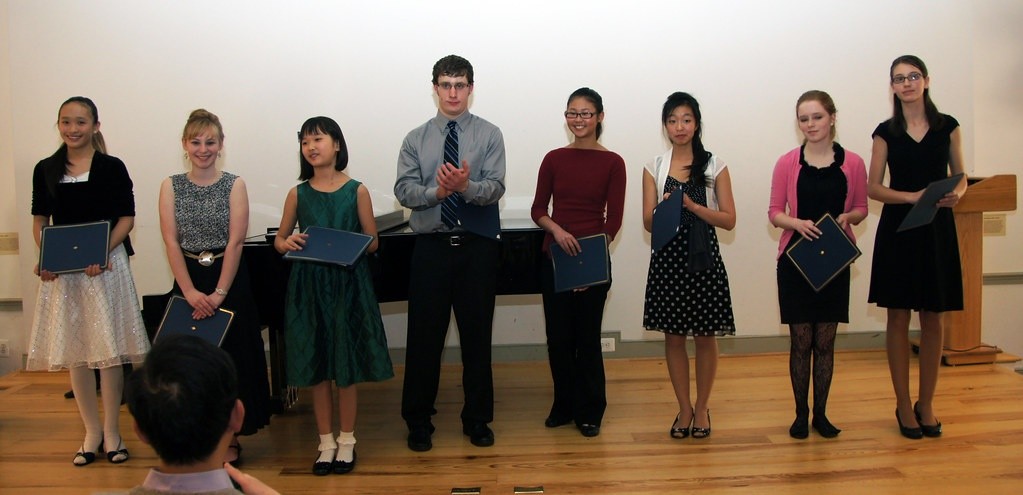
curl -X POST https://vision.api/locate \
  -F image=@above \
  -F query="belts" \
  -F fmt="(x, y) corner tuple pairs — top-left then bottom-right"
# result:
(421, 232), (491, 247)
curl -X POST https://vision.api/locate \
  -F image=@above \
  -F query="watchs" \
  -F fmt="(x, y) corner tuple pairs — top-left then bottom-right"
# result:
(215, 287), (228, 296)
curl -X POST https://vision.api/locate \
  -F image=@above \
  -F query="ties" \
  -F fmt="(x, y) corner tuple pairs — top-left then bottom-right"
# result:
(441, 121), (459, 230)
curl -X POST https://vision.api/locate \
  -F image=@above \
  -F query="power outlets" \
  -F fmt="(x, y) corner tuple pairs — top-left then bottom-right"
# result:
(601, 337), (616, 353)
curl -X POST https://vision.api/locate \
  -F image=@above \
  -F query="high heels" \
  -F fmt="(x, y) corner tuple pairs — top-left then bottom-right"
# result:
(73, 433), (129, 466)
(671, 408), (711, 438)
(896, 401), (942, 439)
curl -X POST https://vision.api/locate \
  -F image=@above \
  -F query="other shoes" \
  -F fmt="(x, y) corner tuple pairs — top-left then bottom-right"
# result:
(312, 449), (357, 476)
(222, 442), (242, 468)
(788, 414), (842, 439)
(64, 388), (76, 399)
(544, 409), (600, 437)
(408, 417), (433, 452)
(462, 418), (495, 446)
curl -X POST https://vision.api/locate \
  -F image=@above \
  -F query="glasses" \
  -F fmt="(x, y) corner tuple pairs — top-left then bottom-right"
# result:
(893, 72), (922, 84)
(439, 82), (471, 89)
(566, 112), (599, 119)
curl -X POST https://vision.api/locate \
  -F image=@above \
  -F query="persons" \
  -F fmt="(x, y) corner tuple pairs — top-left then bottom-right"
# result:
(274, 116), (394, 475)
(135, 109), (274, 467)
(394, 55), (506, 452)
(643, 92), (736, 439)
(129, 333), (282, 495)
(531, 87), (626, 437)
(767, 90), (867, 439)
(867, 55), (968, 438)
(24, 97), (151, 467)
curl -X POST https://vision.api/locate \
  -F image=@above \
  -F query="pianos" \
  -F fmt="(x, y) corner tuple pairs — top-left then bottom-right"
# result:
(139, 210), (550, 416)
